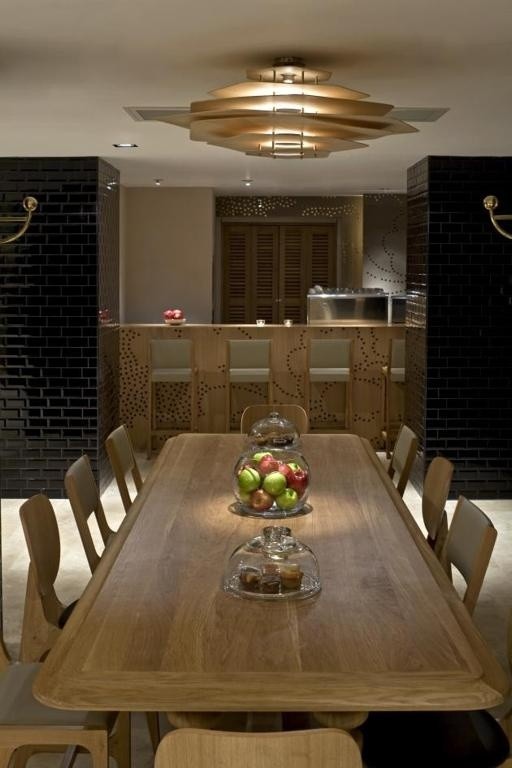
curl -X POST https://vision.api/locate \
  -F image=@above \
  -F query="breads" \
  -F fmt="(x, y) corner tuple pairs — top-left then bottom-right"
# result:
(240, 563), (304, 594)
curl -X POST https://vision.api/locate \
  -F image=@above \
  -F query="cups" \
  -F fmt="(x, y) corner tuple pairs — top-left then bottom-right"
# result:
(256, 320), (266, 327)
(284, 320), (293, 327)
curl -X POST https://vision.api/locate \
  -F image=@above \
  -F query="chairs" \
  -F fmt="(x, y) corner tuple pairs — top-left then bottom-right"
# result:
(432, 494), (493, 619)
(383, 422), (415, 499)
(4, 639), (138, 765)
(104, 422), (148, 512)
(414, 453), (460, 586)
(60, 451), (119, 576)
(236, 401), (310, 432)
(148, 726), (364, 768)
(9, 491), (96, 665)
(1, 721), (113, 767)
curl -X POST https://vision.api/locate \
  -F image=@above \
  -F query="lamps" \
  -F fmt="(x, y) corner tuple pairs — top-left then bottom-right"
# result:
(157, 58), (418, 158)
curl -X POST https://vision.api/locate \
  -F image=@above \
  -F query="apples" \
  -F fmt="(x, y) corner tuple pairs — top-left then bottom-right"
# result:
(163, 309), (183, 319)
(236, 452), (310, 511)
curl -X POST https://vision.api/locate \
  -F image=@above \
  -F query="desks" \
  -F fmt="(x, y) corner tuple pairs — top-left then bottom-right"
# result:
(33, 429), (511, 742)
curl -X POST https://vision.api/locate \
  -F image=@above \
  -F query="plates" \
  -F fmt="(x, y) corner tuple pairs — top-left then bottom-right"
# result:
(164, 319), (186, 324)
(225, 570), (321, 599)
